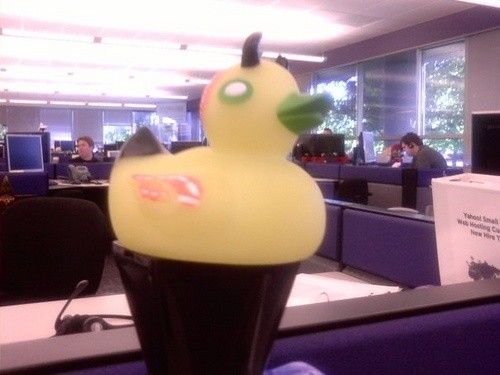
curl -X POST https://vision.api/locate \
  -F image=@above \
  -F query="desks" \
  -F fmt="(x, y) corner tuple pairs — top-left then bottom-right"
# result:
(297, 162), (464, 212)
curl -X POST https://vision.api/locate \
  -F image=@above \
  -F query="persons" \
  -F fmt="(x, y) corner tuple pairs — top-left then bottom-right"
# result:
(400, 133), (447, 169)
(69, 136), (102, 163)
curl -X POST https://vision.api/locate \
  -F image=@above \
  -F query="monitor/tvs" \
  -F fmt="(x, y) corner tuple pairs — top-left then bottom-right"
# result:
(469, 112), (500, 173)
(298, 133), (344, 159)
(170, 142), (202, 154)
(61, 141), (75, 152)
(104, 141), (124, 155)
(3, 132), (52, 163)
(2, 135), (46, 172)
(358, 131), (377, 164)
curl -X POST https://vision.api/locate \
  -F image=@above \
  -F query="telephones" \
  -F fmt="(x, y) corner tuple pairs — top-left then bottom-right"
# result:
(68, 164), (92, 183)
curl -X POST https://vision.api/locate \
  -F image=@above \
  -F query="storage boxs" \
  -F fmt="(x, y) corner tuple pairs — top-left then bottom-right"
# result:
(432, 174), (500, 283)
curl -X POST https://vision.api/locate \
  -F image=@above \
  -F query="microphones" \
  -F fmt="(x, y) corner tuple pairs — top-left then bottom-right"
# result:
(55, 280), (88, 328)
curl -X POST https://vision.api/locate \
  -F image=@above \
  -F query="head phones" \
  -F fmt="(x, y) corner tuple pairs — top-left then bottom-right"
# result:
(57, 314), (136, 335)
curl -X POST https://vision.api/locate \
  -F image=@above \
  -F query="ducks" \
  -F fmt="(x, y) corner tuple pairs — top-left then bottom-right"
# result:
(106, 28), (337, 266)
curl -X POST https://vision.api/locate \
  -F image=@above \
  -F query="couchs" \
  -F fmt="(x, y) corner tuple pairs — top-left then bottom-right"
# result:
(315, 199), (439, 287)
(0, 285), (499, 375)
(0, 197), (115, 297)
